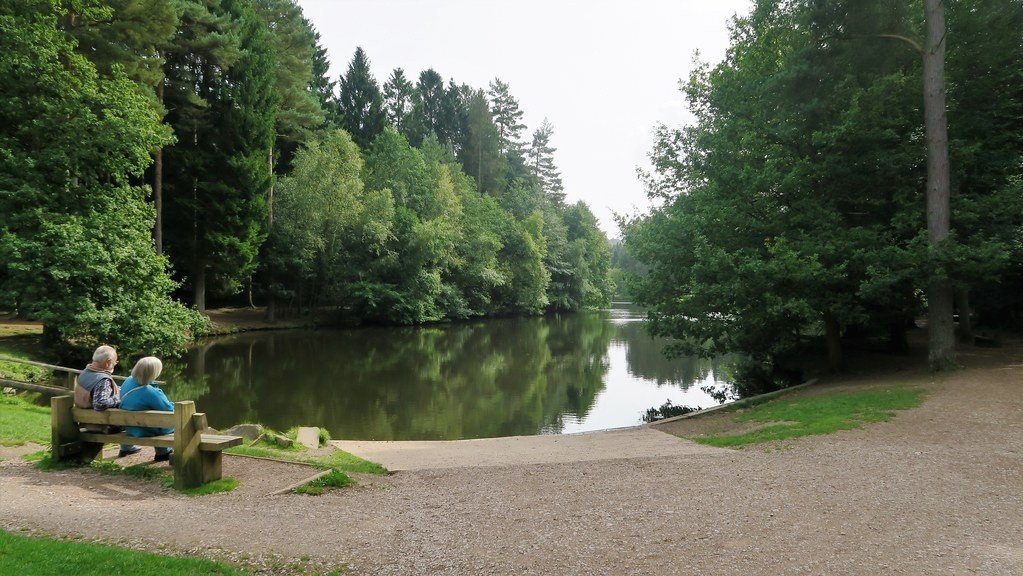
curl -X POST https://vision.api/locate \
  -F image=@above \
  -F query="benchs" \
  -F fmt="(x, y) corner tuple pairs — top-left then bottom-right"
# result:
(50, 396), (244, 491)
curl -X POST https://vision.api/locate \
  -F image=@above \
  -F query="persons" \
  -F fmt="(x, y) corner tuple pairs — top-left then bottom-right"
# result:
(119, 356), (174, 437)
(74, 346), (173, 461)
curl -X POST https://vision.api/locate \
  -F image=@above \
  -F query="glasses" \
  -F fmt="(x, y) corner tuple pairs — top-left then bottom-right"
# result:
(111, 360), (119, 365)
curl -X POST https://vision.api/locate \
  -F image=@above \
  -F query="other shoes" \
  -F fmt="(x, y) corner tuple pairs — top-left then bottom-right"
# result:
(155, 451), (173, 461)
(119, 447), (140, 457)
